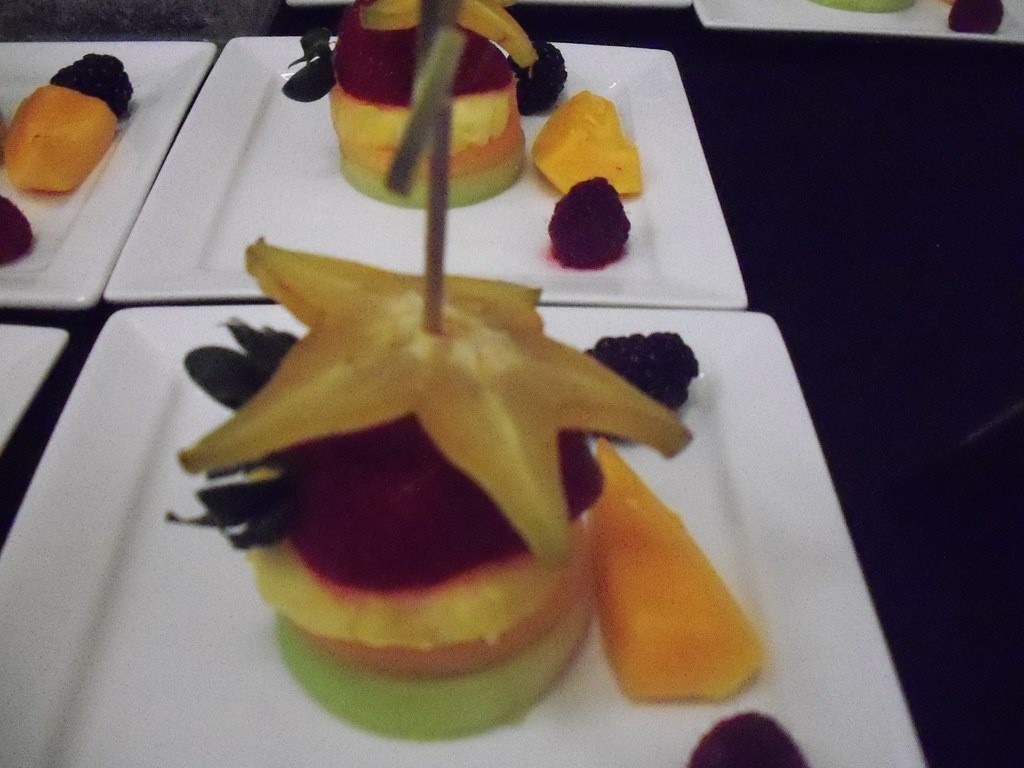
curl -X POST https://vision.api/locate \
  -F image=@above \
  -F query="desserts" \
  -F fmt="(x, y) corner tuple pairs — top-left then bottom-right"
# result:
(282, 0), (645, 269)
(180, 230), (810, 768)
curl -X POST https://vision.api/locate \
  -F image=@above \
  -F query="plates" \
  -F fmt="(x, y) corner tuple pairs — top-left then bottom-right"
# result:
(1, 325), (72, 453)
(100, 36), (747, 313)
(0, 40), (220, 311)
(692, 0), (1023, 46)
(286, 0), (692, 8)
(0, 306), (930, 767)
